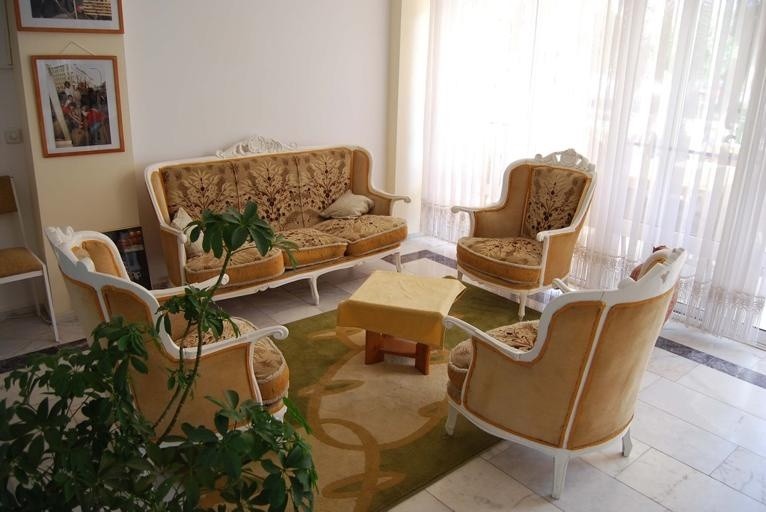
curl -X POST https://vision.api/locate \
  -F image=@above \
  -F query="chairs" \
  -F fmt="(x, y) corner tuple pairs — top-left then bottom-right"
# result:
(43, 224), (289, 449)
(452, 148), (597, 321)
(441, 246), (688, 500)
(0, 174), (59, 342)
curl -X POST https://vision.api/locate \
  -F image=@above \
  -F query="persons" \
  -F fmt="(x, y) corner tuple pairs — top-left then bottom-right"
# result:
(61, 80), (111, 147)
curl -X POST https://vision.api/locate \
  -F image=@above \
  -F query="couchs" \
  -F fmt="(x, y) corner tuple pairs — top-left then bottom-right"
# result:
(143, 134), (412, 306)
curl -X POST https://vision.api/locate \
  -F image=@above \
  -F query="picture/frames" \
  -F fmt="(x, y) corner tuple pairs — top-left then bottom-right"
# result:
(31, 55), (124, 157)
(14, 0), (124, 34)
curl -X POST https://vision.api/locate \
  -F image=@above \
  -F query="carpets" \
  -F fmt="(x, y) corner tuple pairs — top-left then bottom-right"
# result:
(194, 275), (568, 512)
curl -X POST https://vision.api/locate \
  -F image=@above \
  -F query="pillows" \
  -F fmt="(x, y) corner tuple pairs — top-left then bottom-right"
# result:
(319, 189), (373, 220)
(172, 207), (206, 257)
